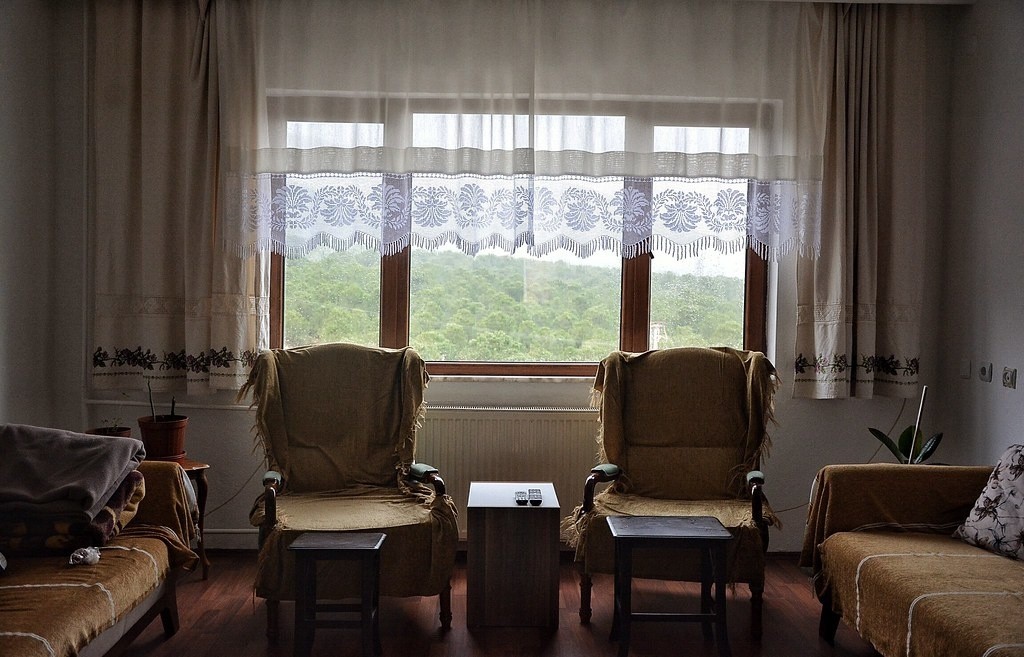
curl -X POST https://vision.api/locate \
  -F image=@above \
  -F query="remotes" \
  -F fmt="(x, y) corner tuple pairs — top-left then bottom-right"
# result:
(528, 489), (542, 506)
(514, 492), (529, 505)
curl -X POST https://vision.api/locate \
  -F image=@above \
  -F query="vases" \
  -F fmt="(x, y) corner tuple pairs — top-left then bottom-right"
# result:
(83, 426), (132, 439)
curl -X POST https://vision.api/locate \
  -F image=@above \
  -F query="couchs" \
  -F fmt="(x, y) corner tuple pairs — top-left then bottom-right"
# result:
(0, 423), (180, 657)
(795, 460), (1024, 657)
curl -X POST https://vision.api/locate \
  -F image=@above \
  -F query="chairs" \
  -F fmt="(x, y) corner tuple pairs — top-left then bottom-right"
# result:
(242, 345), (459, 641)
(565, 345), (780, 623)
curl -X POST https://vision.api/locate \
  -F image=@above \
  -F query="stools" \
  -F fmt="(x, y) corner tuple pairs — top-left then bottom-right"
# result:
(286, 533), (387, 657)
(605, 515), (735, 657)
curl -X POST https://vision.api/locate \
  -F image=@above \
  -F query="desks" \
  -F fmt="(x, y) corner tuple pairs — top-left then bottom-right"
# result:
(466, 480), (563, 631)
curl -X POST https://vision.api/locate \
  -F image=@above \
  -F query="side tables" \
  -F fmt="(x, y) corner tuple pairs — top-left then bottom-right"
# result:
(141, 458), (214, 582)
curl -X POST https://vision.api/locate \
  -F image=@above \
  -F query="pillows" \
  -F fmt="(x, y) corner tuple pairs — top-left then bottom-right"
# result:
(948, 443), (1024, 561)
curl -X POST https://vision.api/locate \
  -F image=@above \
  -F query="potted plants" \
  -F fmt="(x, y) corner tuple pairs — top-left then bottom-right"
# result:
(90, 341), (244, 462)
